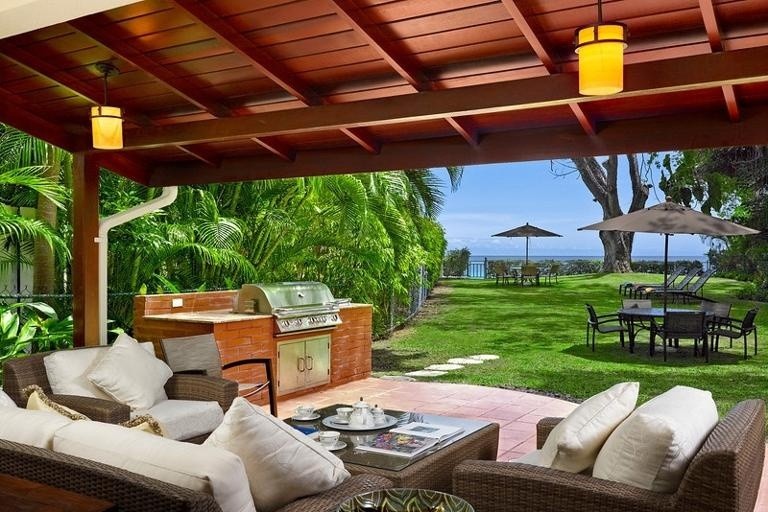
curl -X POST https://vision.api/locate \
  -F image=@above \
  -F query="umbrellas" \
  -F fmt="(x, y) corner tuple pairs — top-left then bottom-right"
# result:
(575, 196), (762, 312)
(490, 221), (566, 265)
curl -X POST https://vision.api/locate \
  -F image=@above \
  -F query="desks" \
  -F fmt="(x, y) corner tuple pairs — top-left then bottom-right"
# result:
(0, 472), (117, 512)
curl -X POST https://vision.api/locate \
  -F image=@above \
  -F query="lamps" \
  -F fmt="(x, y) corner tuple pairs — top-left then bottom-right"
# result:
(88, 61), (127, 151)
(574, 0), (629, 96)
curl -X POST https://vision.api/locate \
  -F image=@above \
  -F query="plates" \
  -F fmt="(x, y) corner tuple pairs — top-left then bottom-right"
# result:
(318, 441), (348, 451)
(292, 414), (321, 420)
(322, 414), (397, 431)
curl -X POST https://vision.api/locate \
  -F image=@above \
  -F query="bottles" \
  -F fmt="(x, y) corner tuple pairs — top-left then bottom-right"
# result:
(350, 397), (386, 428)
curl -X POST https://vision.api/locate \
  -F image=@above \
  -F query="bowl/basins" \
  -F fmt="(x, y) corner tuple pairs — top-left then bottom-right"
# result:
(337, 487), (473, 511)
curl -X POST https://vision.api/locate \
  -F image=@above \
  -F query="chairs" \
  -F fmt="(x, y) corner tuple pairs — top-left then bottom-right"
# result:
(583, 264), (760, 364)
(159, 332), (278, 418)
(2, 338), (239, 446)
(451, 398), (767, 512)
(493, 259), (561, 287)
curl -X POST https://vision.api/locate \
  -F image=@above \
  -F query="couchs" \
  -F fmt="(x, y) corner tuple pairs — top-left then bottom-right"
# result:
(0, 416), (395, 512)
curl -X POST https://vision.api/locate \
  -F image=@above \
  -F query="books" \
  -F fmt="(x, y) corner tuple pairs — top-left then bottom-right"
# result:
(354, 421), (466, 458)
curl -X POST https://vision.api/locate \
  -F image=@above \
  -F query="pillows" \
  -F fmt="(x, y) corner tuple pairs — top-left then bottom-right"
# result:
(592, 384), (720, 494)
(86, 332), (175, 414)
(539, 380), (640, 473)
(52, 421), (257, 512)
(201, 395), (352, 512)
(22, 384), (93, 421)
(116, 415), (165, 437)
(42, 340), (170, 421)
(0, 406), (73, 451)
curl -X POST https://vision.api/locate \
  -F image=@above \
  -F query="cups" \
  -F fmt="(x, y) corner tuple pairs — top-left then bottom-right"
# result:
(297, 406), (315, 418)
(319, 431), (340, 447)
(336, 408), (353, 419)
